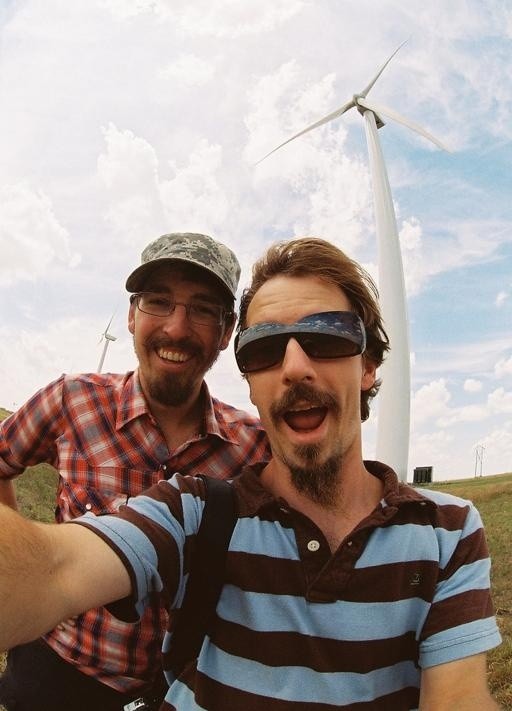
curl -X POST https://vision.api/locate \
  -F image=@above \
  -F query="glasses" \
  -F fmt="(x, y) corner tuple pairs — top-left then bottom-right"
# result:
(235, 310), (367, 373)
(133, 287), (226, 328)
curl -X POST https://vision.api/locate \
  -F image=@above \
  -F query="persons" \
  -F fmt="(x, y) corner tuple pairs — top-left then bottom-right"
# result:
(0, 236), (502, 710)
(0, 232), (269, 706)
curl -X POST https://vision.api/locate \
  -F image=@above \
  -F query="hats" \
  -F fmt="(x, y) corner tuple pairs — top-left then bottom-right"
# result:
(125, 232), (239, 304)
